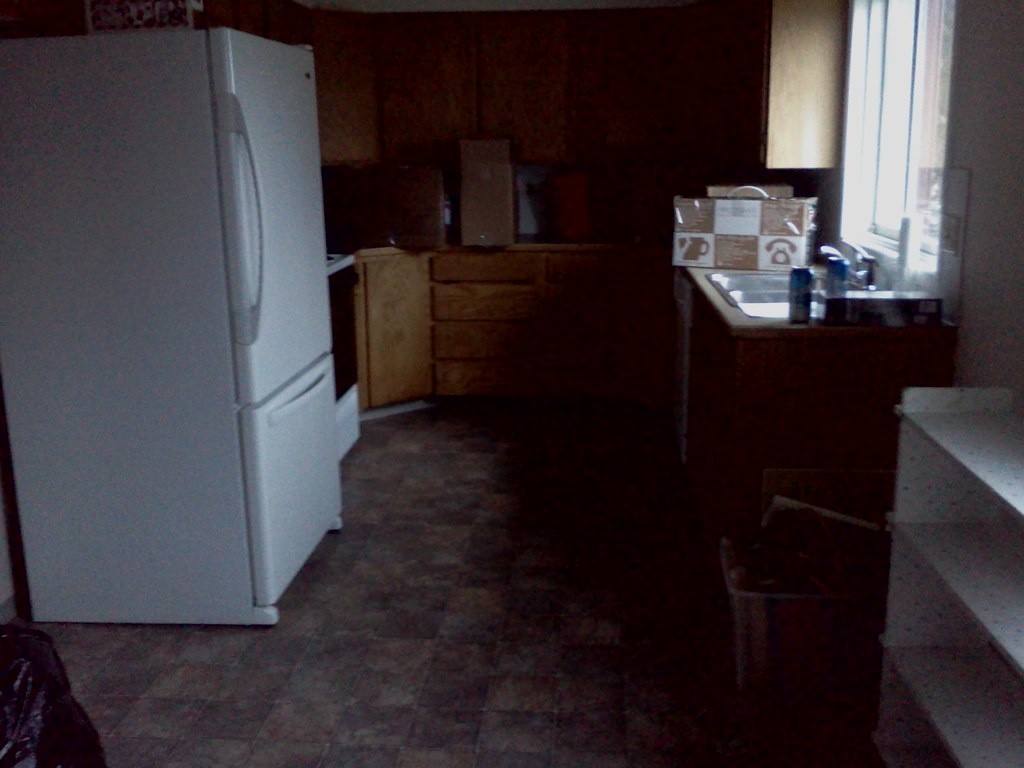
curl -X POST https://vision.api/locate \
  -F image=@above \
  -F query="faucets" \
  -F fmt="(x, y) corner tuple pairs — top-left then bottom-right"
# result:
(817, 237), (877, 290)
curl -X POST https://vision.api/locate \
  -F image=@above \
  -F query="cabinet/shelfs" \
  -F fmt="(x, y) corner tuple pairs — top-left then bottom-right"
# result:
(872, 386), (1024, 768)
(745, 0), (846, 171)
(347, 240), (961, 469)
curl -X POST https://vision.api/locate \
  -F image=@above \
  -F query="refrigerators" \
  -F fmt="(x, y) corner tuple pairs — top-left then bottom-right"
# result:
(0, 28), (343, 624)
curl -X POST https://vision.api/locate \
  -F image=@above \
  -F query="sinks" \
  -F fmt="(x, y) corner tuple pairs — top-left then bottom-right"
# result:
(707, 271), (821, 291)
(723, 291), (824, 318)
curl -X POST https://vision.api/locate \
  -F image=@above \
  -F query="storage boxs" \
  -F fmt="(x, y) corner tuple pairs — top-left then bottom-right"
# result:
(442, 139), (515, 247)
(719, 532), (887, 706)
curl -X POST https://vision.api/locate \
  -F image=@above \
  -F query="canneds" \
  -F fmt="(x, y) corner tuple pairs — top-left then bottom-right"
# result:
(826, 257), (850, 290)
(788, 266), (814, 323)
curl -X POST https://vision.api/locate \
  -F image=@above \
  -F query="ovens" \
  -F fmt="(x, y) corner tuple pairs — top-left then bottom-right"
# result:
(327, 254), (361, 459)
